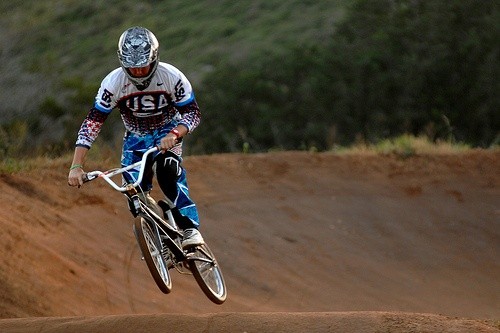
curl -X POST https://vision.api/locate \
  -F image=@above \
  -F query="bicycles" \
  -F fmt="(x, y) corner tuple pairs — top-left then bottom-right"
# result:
(66, 143), (228, 305)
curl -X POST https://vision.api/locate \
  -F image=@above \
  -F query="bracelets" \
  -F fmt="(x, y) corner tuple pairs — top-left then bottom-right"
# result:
(68, 164), (83, 170)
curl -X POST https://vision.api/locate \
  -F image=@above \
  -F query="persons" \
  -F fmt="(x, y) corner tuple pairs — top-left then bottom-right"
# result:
(68, 25), (207, 249)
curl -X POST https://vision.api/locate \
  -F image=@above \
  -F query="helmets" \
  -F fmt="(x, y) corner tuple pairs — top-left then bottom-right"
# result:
(117, 26), (159, 87)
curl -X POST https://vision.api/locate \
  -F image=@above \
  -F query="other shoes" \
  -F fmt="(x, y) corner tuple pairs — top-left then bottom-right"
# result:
(162, 245), (174, 269)
(182, 228), (205, 250)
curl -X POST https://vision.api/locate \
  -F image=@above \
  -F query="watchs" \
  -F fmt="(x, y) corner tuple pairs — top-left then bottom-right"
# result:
(169, 129), (180, 139)
